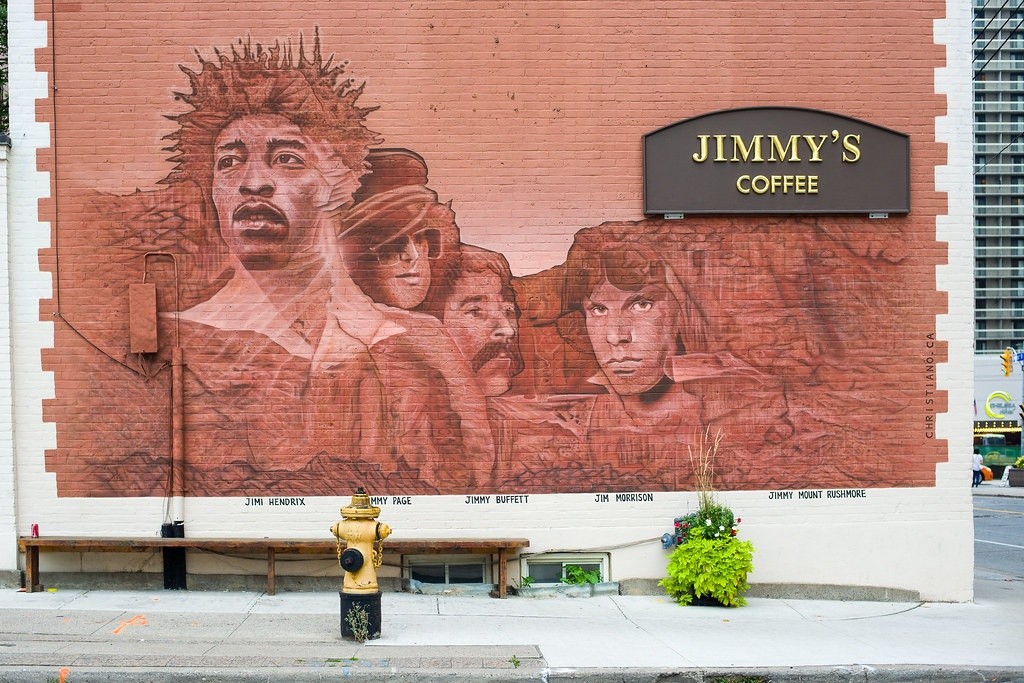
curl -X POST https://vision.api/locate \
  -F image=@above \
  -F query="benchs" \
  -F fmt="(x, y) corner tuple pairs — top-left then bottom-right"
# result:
(18, 535), (530, 599)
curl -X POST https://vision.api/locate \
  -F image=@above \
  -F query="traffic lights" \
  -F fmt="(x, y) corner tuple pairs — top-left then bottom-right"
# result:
(1000, 349), (1012, 377)
(1019, 403), (1024, 423)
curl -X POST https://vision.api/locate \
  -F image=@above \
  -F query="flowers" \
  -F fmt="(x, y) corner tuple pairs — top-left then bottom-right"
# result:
(674, 505), (743, 550)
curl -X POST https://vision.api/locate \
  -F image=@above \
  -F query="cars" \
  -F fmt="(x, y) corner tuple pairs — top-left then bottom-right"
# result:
(973, 466), (994, 485)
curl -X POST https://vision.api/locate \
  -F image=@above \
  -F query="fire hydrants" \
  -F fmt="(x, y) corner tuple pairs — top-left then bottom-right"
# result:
(326, 487), (393, 642)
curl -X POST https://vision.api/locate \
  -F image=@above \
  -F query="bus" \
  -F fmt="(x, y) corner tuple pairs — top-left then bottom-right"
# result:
(974, 434), (1006, 456)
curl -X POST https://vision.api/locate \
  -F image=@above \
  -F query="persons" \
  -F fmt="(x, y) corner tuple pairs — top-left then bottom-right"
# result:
(972, 449), (984, 488)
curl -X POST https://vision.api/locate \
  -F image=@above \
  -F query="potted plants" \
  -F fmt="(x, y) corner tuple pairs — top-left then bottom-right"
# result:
(1009, 455), (1024, 487)
